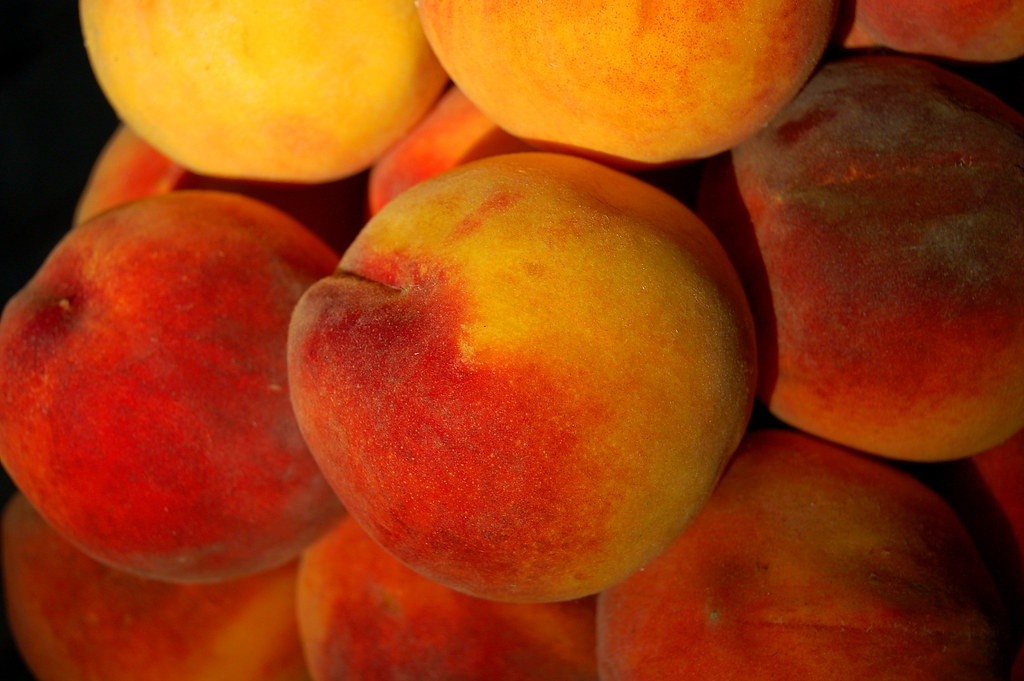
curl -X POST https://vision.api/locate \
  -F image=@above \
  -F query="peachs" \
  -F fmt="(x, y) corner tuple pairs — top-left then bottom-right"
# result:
(0, 0), (1024, 681)
